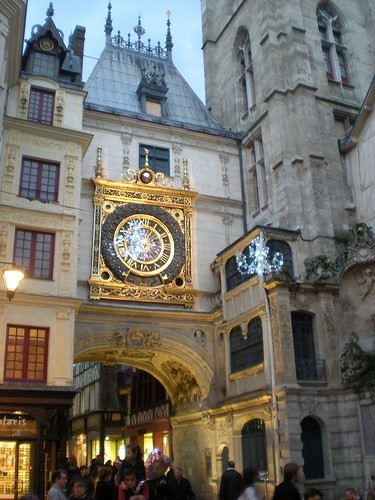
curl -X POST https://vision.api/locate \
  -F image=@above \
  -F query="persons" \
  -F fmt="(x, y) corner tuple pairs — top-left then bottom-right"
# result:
(46, 442), (197, 500)
(234, 467), (266, 500)
(219, 461), (245, 500)
(304, 487), (324, 500)
(344, 487), (363, 500)
(271, 462), (302, 500)
(365, 472), (375, 500)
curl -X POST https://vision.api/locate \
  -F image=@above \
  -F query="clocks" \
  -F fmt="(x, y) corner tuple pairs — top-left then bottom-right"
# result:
(114, 214), (174, 277)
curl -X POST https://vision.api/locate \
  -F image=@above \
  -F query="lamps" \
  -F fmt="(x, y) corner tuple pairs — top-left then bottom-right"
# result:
(0, 261), (24, 301)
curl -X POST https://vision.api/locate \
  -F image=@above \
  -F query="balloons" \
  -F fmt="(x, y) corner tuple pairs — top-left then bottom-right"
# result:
(146, 448), (170, 480)
